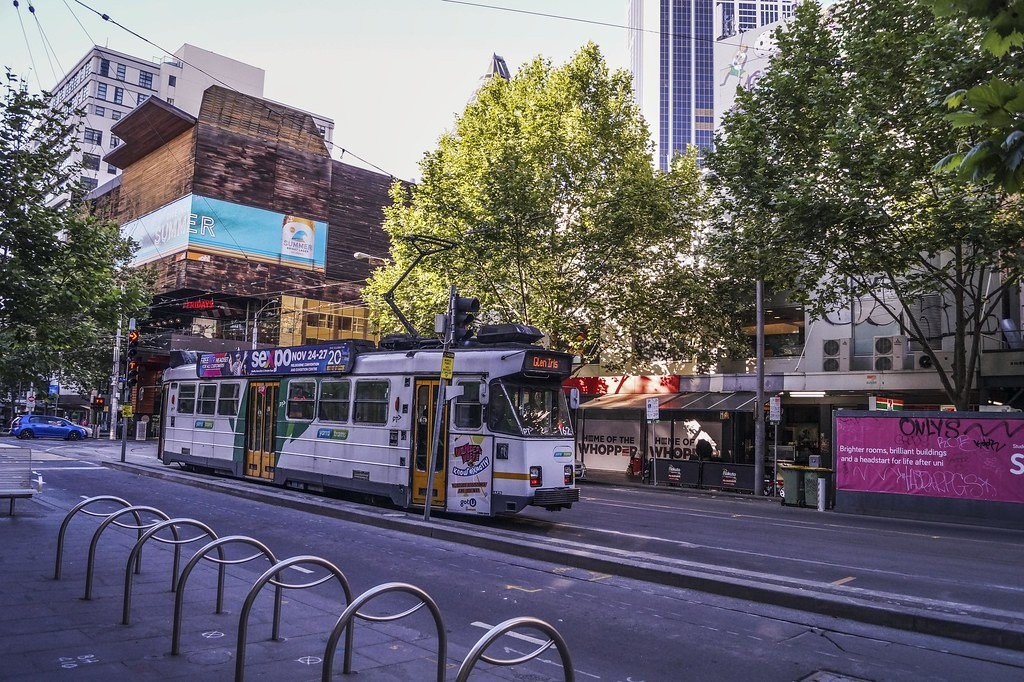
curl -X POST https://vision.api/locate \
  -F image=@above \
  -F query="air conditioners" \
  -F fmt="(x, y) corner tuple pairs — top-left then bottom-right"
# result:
(873, 356), (903, 371)
(914, 351), (950, 371)
(873, 335), (907, 355)
(822, 338), (855, 357)
(822, 357), (851, 372)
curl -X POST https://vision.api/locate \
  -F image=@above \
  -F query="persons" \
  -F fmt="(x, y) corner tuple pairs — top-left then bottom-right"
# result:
(696, 439), (713, 461)
(493, 406), (521, 433)
(80, 417), (89, 426)
(765, 449), (778, 496)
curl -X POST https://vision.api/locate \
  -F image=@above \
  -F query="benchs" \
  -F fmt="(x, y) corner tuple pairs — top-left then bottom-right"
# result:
(0, 447), (47, 516)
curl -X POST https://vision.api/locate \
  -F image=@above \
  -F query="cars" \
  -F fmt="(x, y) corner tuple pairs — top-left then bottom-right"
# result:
(8, 415), (87, 441)
(30, 416), (94, 440)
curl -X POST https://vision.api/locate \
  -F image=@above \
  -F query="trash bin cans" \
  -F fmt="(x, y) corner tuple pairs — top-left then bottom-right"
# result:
(780, 465), (804, 508)
(801, 467), (835, 509)
(136, 420), (146, 440)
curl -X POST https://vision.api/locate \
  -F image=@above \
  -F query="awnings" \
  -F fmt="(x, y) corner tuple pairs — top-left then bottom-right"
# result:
(554, 393), (781, 414)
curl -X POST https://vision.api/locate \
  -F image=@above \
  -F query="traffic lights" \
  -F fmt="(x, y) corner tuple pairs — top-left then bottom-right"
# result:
(127, 360), (140, 388)
(127, 328), (139, 357)
(446, 291), (481, 343)
(93, 396), (105, 412)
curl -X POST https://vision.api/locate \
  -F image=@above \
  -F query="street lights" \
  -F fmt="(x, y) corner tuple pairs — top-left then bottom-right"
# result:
(251, 299), (280, 349)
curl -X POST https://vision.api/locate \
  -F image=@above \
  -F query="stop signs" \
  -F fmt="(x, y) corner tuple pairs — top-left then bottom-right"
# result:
(28, 396), (35, 402)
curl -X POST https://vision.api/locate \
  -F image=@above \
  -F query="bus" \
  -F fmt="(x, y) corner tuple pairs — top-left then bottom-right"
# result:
(149, 232), (586, 519)
